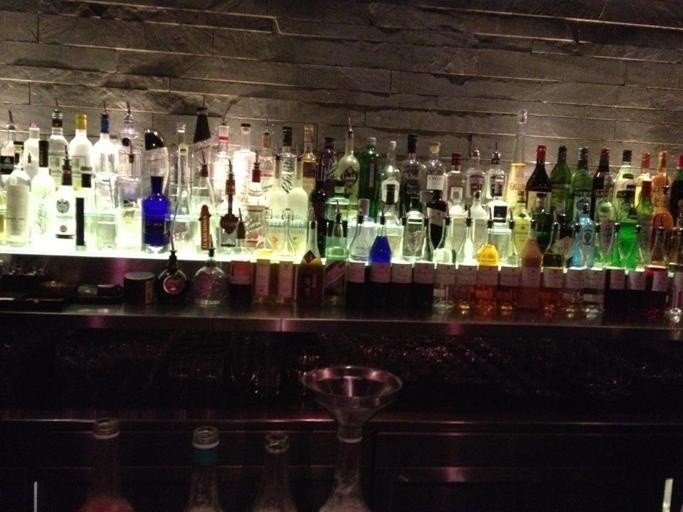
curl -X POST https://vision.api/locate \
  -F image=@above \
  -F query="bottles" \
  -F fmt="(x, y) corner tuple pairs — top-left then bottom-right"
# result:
(185, 427), (225, 509)
(81, 417), (132, 505)
(318, 425), (374, 511)
(0, 106), (681, 326)
(251, 431), (293, 510)
